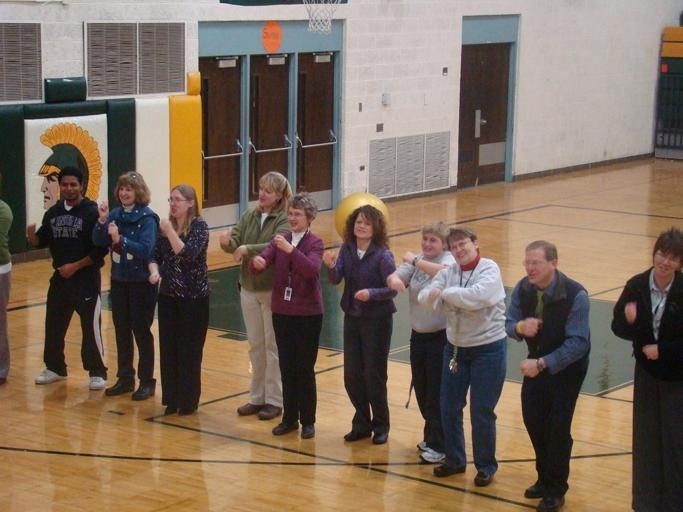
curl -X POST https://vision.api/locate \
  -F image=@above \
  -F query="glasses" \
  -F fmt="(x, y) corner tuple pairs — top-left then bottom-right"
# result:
(168, 198), (190, 203)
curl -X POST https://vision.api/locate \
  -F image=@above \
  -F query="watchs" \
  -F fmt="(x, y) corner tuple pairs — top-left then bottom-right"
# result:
(536, 358), (543, 372)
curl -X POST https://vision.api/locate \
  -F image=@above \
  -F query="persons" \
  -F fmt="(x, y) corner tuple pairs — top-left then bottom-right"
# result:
(217, 170), (294, 419)
(321, 205), (400, 445)
(145, 184), (211, 417)
(418, 229), (507, 487)
(39, 170), (61, 209)
(249, 189), (325, 439)
(92, 172), (160, 401)
(28, 165), (110, 392)
(611, 224), (683, 512)
(384, 222), (450, 464)
(0, 199), (16, 386)
(505, 239), (591, 512)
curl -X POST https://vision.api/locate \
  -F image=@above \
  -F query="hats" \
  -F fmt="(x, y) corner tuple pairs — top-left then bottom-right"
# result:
(39, 123), (102, 201)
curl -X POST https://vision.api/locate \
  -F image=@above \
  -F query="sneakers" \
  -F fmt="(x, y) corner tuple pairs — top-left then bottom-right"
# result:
(418, 440), (446, 462)
(35, 369), (67, 384)
(89, 376), (106, 389)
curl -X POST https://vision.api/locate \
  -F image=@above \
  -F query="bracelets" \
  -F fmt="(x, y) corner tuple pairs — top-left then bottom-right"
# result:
(516, 321), (526, 335)
(411, 256), (420, 264)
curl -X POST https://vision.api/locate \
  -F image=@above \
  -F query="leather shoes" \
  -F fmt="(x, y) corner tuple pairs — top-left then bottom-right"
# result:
(536, 494), (564, 510)
(373, 432), (388, 443)
(344, 430), (372, 441)
(105, 377), (135, 395)
(132, 378), (156, 399)
(434, 464), (466, 477)
(524, 482), (548, 497)
(475, 472), (494, 486)
(164, 405), (198, 415)
(238, 403), (282, 419)
(272, 419), (315, 440)
